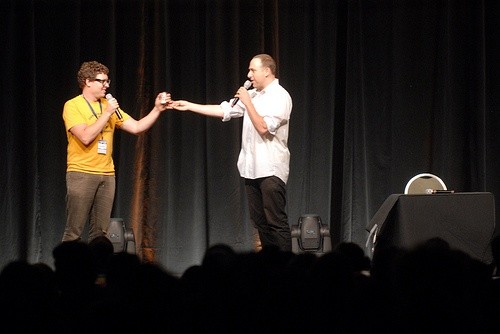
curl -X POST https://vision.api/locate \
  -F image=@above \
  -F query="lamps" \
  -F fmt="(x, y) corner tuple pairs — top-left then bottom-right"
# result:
(291, 214), (330, 252)
(106, 218), (134, 253)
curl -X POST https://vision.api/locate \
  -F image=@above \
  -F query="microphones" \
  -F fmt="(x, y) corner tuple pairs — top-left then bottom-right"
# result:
(425, 189), (454, 195)
(231, 80), (251, 108)
(106, 94), (122, 119)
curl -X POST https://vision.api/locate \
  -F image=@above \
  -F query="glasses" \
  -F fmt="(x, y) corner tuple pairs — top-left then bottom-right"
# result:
(91, 78), (111, 84)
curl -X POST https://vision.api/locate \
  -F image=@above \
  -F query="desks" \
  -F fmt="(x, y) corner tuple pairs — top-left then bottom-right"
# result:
(364, 192), (495, 264)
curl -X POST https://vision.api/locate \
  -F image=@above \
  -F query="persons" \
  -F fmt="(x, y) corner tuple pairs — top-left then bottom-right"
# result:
(167, 54), (293, 252)
(0, 236), (500, 334)
(62, 61), (172, 244)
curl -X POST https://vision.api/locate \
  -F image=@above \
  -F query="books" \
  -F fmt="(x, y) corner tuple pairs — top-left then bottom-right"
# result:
(426, 189), (455, 195)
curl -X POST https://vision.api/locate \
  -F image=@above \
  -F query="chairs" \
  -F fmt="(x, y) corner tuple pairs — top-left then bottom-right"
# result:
(403, 173), (447, 194)
